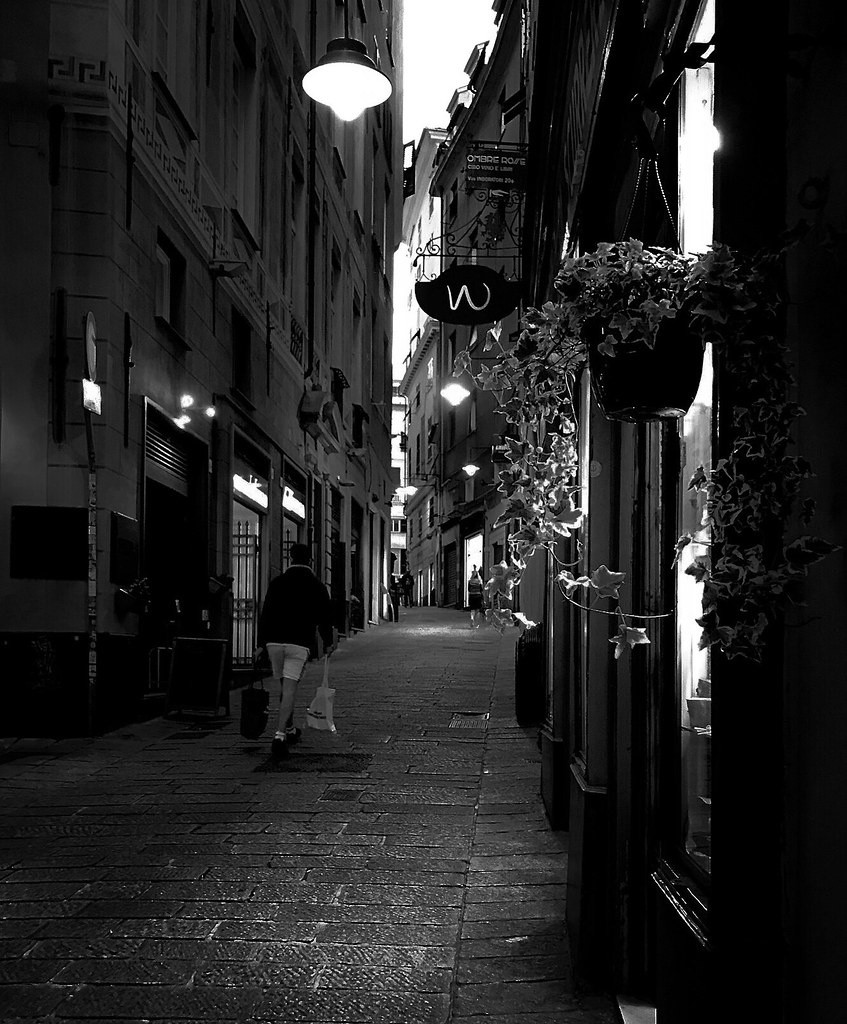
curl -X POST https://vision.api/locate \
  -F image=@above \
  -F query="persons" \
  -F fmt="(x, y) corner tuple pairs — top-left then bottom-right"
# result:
(259, 543), (335, 762)
(388, 581), (400, 622)
(400, 570), (414, 608)
(468, 571), (483, 628)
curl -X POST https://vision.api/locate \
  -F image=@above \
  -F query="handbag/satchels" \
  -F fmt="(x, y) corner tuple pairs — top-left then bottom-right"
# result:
(239, 658), (271, 740)
(305, 654), (340, 735)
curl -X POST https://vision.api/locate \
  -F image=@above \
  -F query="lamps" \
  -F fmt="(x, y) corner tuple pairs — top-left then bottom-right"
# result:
(461, 445), (492, 477)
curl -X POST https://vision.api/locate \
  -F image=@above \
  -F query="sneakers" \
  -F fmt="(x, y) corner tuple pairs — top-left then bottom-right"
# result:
(286, 728), (302, 740)
(270, 739), (287, 755)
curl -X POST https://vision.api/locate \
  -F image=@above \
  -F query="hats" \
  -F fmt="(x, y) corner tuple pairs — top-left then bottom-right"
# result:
(290, 544), (313, 560)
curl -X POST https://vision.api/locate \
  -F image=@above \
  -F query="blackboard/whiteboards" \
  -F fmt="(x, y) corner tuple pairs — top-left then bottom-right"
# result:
(165, 636), (227, 713)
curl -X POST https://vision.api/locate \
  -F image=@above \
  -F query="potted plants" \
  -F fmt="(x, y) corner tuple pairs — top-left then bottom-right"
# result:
(474, 238), (842, 675)
